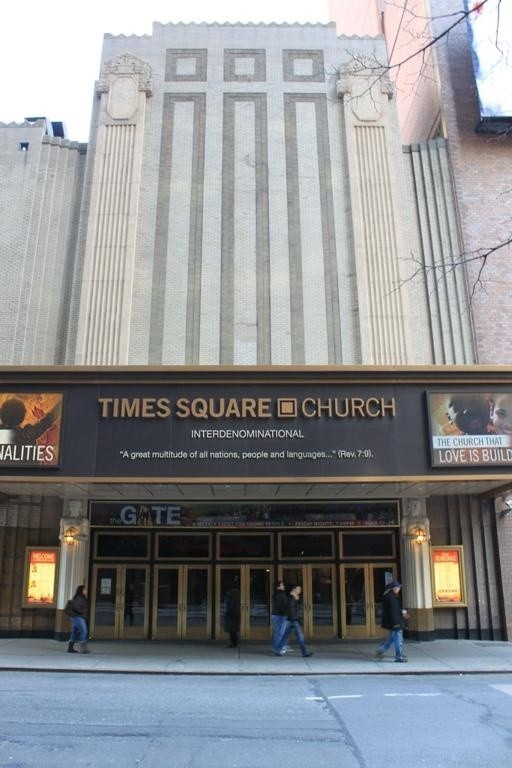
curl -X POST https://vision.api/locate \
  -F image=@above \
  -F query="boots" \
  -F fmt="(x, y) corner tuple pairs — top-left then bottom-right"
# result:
(80, 640), (89, 653)
(67, 641), (77, 653)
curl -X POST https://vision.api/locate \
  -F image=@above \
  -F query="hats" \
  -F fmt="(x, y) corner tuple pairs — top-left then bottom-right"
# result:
(389, 581), (403, 588)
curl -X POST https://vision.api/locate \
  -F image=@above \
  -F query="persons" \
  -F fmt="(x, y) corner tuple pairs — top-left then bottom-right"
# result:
(376, 582), (410, 662)
(445, 394), (496, 435)
(489, 393), (512, 435)
(275, 585), (312, 657)
(64, 585), (91, 654)
(272, 581), (295, 653)
(223, 581), (241, 648)
(0, 398), (62, 465)
(124, 580), (134, 626)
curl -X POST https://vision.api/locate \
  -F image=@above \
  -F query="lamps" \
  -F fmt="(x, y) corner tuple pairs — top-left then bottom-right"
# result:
(63, 526), (78, 544)
(410, 526), (426, 544)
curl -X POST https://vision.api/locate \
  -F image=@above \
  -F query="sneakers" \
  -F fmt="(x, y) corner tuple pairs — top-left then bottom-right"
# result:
(396, 657), (407, 662)
(302, 652), (313, 657)
(272, 645), (294, 655)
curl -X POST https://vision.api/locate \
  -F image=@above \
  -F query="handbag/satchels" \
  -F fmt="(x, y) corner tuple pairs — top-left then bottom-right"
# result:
(64, 600), (74, 617)
